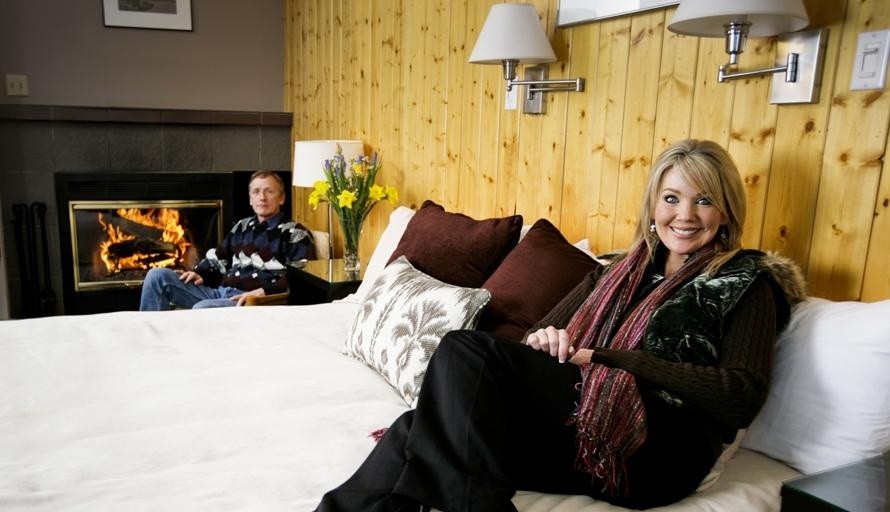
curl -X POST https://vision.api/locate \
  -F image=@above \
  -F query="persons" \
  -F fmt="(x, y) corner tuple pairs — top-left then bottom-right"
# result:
(138, 169), (317, 312)
(312, 137), (803, 512)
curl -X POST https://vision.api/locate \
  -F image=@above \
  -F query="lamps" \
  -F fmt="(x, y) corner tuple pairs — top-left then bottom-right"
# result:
(468, 3), (585, 115)
(292, 141), (365, 259)
(667, 0), (830, 106)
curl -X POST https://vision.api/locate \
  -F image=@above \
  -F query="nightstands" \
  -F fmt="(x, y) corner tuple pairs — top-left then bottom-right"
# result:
(779, 452), (890, 512)
(286, 257), (368, 305)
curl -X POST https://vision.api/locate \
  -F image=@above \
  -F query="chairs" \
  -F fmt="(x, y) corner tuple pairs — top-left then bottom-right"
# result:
(169, 230), (329, 310)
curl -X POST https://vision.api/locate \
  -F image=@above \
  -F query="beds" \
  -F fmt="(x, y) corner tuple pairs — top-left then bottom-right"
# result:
(0, 297), (890, 512)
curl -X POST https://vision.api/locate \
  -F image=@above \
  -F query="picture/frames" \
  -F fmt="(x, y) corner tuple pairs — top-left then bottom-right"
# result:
(101, 0), (194, 32)
(555, 0), (680, 29)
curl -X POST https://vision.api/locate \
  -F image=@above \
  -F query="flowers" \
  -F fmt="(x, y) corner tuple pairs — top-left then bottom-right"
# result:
(308, 143), (397, 266)
